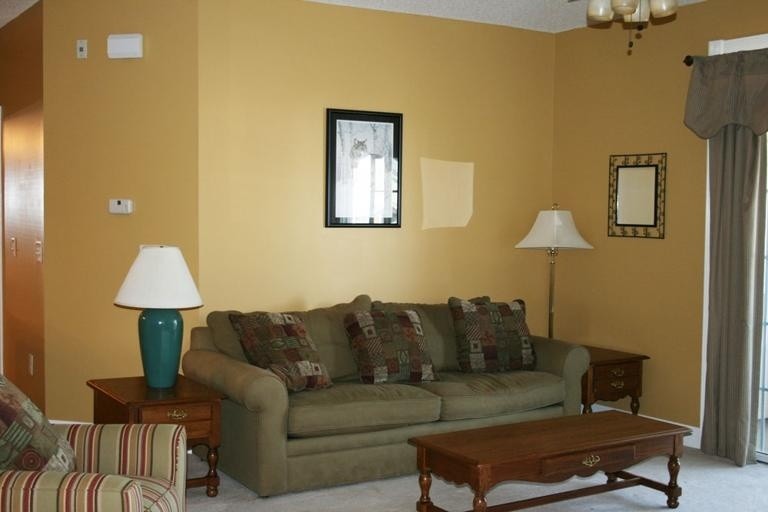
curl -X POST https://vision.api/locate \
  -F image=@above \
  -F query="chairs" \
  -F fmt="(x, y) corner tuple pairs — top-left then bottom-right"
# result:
(1, 374), (187, 512)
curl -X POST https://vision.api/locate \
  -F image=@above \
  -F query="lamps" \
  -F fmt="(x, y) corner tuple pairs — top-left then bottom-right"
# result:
(111, 245), (203, 390)
(586, 0), (678, 47)
(515, 203), (595, 339)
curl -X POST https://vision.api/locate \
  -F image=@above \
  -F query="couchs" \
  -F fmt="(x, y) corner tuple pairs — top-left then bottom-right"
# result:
(182, 297), (592, 499)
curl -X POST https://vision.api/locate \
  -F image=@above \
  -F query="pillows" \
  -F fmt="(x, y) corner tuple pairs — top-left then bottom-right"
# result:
(1, 372), (78, 473)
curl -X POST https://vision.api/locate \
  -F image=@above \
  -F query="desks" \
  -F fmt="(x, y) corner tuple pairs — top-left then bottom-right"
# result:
(582, 344), (650, 415)
(85, 374), (227, 498)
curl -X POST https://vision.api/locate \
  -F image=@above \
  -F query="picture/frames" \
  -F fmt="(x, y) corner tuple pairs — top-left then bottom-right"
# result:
(608, 153), (667, 239)
(324, 107), (403, 228)
